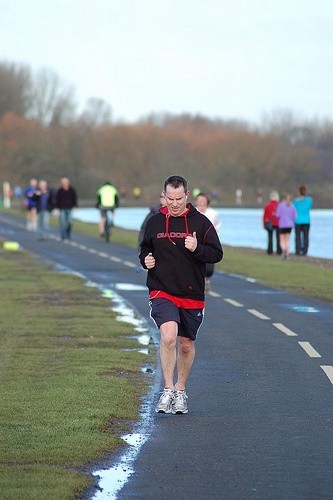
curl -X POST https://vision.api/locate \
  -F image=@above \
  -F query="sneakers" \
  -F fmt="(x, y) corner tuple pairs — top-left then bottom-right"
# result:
(155, 388), (176, 414)
(170, 387), (189, 414)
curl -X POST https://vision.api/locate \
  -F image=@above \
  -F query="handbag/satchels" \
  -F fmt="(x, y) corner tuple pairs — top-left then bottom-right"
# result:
(264, 222), (272, 230)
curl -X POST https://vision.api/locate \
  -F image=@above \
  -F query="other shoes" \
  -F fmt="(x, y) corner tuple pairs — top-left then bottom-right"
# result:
(205, 278), (211, 292)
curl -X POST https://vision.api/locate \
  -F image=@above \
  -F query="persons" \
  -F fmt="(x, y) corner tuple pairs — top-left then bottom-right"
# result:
(96, 181), (118, 238)
(14, 185), (22, 208)
(194, 193), (221, 292)
(139, 174), (223, 414)
(292, 186), (312, 255)
(24, 179), (56, 241)
(138, 191), (166, 250)
(54, 177), (77, 241)
(4, 183), (12, 209)
(275, 192), (296, 260)
(263, 192), (282, 256)
